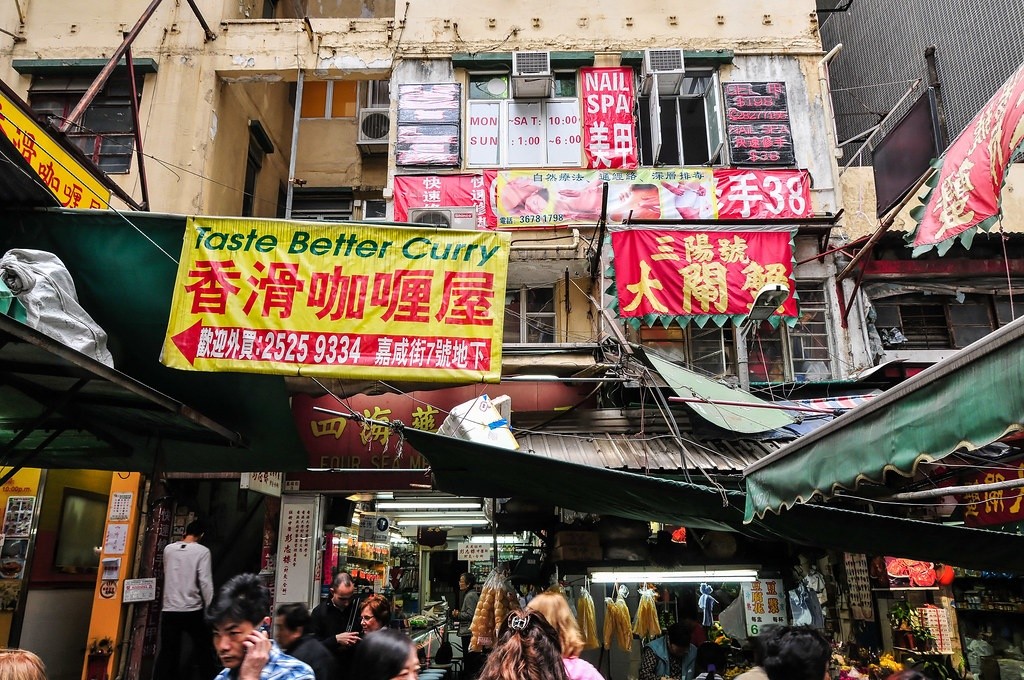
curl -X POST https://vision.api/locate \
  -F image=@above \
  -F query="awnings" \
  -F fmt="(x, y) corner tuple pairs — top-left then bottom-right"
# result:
(0, 208), (1024, 570)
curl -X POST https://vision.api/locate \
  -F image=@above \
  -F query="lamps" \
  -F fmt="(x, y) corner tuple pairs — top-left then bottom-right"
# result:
(371, 499), (484, 511)
(740, 282), (790, 336)
(468, 536), (519, 543)
(376, 510), (486, 518)
(587, 563), (762, 584)
(410, 475), (432, 488)
(394, 519), (490, 526)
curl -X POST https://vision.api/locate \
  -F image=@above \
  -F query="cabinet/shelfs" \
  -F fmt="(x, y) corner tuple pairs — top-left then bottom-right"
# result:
(468, 542), (533, 583)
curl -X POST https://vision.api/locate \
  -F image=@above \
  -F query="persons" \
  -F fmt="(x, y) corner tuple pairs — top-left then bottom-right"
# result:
(479, 592), (832, 680)
(501, 178), (707, 222)
(0, 521), (475, 680)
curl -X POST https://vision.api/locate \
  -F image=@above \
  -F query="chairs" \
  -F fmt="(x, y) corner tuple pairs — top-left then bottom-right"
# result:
(748, 351), (769, 382)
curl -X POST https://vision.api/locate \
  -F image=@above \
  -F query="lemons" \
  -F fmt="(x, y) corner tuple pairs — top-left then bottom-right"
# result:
(870, 654), (902, 672)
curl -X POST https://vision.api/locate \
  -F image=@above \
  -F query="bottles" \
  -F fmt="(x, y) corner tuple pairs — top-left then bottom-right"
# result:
(344, 537), (401, 595)
(471, 563), (495, 583)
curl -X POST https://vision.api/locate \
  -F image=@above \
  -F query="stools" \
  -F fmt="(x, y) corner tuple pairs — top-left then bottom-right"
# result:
(415, 658), (462, 680)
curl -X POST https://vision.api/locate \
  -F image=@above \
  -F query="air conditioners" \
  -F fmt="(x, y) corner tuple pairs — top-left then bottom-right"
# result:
(362, 198), (387, 221)
(408, 205), (476, 231)
(698, 346), (731, 379)
(357, 108), (390, 156)
(511, 50), (553, 99)
(641, 47), (685, 96)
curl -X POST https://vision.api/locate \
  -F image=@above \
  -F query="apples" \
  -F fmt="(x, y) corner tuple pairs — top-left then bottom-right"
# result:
(410, 620), (427, 625)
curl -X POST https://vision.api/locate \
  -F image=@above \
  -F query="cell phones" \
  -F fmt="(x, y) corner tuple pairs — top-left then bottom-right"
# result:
(244, 623), (271, 653)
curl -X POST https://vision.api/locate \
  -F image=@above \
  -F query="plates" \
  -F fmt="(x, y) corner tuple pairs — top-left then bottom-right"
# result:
(0, 561), (23, 578)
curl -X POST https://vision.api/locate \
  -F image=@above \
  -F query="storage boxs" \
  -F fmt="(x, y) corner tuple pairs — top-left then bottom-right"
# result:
(436, 393), (519, 452)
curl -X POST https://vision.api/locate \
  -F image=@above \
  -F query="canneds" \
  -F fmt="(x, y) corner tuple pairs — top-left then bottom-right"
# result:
(988, 595), (1023, 611)
